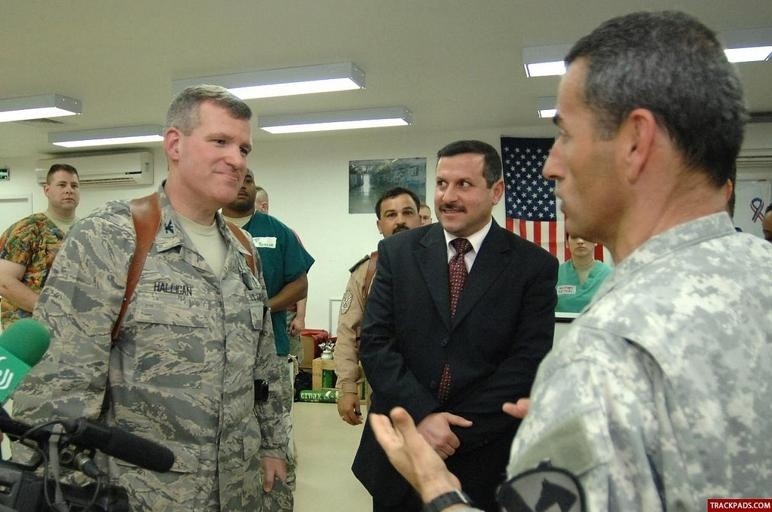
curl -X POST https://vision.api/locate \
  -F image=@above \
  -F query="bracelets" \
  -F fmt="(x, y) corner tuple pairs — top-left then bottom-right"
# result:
(418, 489), (474, 511)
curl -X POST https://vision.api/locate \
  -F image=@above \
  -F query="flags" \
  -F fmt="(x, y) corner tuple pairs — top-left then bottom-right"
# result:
(499, 132), (605, 266)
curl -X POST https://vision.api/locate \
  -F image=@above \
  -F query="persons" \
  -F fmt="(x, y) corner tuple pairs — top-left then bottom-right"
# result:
(761, 202), (772, 240)
(220, 163), (314, 357)
(369, 10), (772, 512)
(334, 185), (420, 424)
(6, 82), (284, 512)
(254, 187), (312, 376)
(1, 164), (82, 321)
(353, 138), (558, 511)
(418, 201), (432, 226)
(555, 228), (613, 314)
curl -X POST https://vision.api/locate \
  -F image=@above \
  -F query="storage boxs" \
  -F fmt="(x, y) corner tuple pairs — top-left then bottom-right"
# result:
(297, 336), (322, 368)
(312, 356), (373, 405)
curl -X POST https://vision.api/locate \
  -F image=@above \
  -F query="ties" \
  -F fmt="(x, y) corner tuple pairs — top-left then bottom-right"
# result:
(435, 237), (472, 406)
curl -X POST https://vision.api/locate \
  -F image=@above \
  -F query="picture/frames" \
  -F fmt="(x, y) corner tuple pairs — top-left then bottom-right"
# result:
(328, 299), (342, 338)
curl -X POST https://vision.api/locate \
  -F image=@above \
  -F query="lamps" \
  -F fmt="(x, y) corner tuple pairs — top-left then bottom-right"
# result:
(522, 24), (771, 78)
(48, 124), (166, 153)
(536, 96), (558, 118)
(0, 92), (81, 123)
(171, 61), (366, 106)
(257, 105), (413, 134)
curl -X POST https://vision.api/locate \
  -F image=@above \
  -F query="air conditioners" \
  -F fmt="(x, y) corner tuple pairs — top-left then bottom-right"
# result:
(35, 151), (153, 188)
(735, 148), (772, 168)
